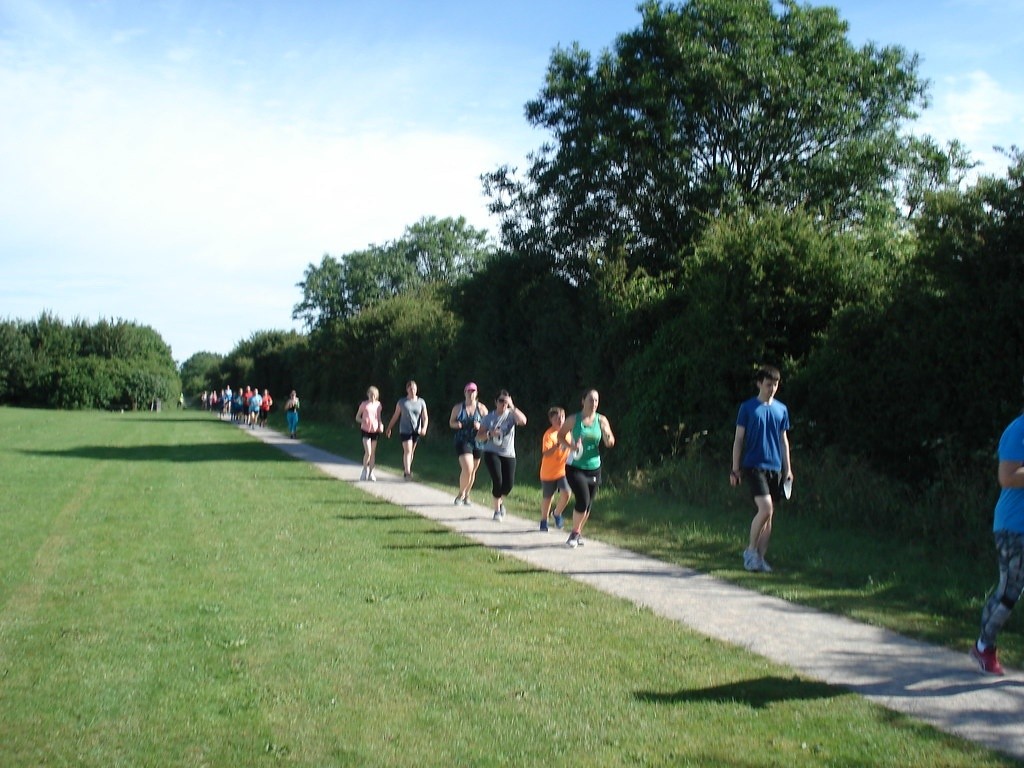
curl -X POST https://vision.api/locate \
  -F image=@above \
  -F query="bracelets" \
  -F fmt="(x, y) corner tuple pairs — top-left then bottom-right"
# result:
(513, 408), (515, 413)
(487, 431), (490, 438)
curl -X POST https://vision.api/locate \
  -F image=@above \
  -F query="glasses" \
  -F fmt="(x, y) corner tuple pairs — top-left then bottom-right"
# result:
(498, 398), (504, 403)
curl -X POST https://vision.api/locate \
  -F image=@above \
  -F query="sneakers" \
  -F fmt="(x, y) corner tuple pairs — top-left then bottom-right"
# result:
(743, 548), (762, 572)
(971, 639), (1006, 676)
(758, 557), (772, 573)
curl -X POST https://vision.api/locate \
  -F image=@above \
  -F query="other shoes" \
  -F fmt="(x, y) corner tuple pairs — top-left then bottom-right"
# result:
(500, 504), (506, 516)
(404, 473), (412, 481)
(454, 494), (465, 506)
(494, 512), (501, 520)
(578, 537), (584, 546)
(551, 510), (562, 530)
(361, 471), (366, 481)
(565, 534), (578, 549)
(540, 520), (549, 531)
(463, 495), (471, 505)
(368, 475), (376, 481)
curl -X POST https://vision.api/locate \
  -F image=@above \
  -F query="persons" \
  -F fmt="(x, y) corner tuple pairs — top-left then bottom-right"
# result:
(475, 389), (527, 521)
(285, 390), (299, 439)
(247, 388), (262, 429)
(972, 413), (1024, 675)
(386, 381), (428, 478)
(259, 389), (272, 428)
(230, 385), (253, 424)
(356, 386), (384, 481)
(540, 408), (572, 531)
(557, 388), (615, 547)
(201, 385), (232, 418)
(450, 383), (488, 506)
(177, 392), (183, 407)
(730, 367), (793, 573)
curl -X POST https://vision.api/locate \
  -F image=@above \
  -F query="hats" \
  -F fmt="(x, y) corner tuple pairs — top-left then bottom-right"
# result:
(464, 383), (477, 391)
